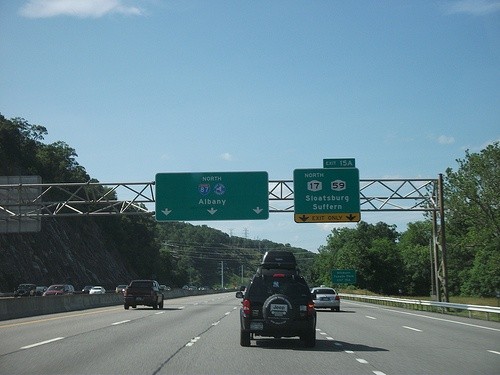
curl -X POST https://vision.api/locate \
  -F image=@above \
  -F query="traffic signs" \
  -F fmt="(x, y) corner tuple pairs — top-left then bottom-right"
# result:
(155, 170), (270, 223)
(293, 168), (364, 224)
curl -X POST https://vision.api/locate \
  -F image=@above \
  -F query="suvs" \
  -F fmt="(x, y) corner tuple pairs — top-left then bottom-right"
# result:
(13, 284), (37, 297)
(235, 250), (318, 344)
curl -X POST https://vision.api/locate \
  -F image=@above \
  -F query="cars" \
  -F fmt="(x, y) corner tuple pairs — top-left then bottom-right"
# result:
(160, 285), (172, 291)
(89, 287), (108, 293)
(82, 286), (92, 294)
(42, 284), (75, 296)
(184, 286), (213, 290)
(124, 279), (165, 309)
(309, 285), (343, 310)
(115, 285), (133, 292)
(36, 287), (48, 295)
